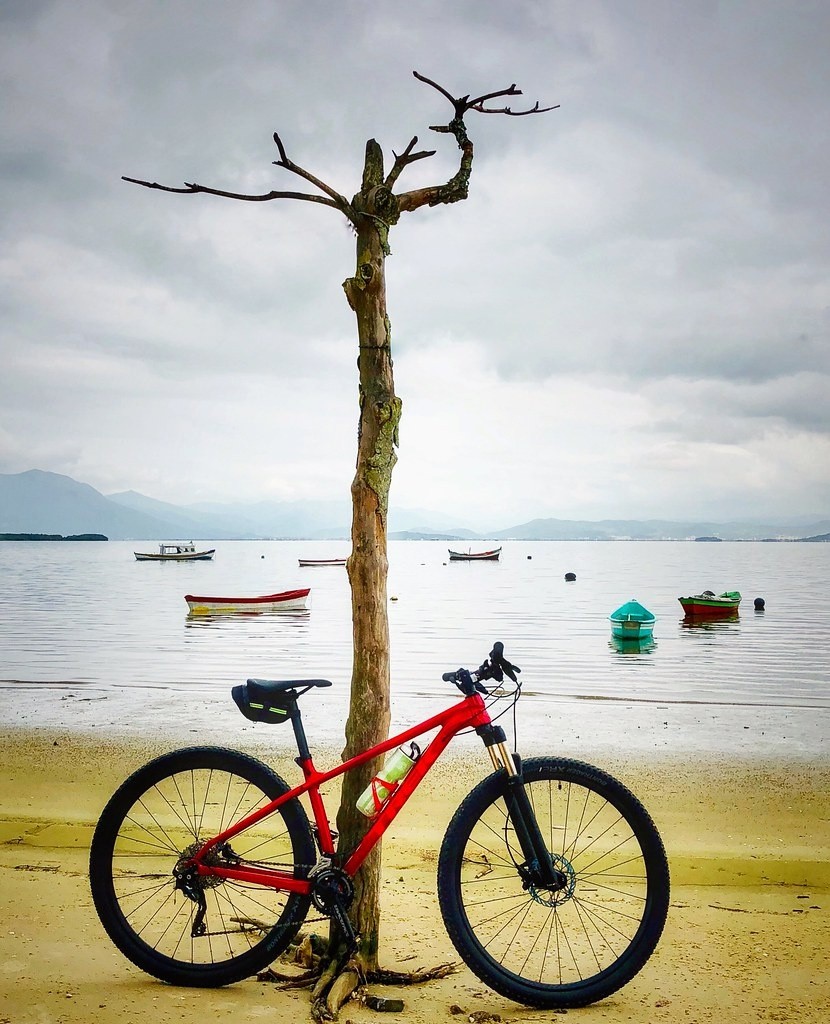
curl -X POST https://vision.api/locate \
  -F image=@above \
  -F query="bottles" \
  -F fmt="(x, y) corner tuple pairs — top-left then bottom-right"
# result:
(356, 739), (420, 817)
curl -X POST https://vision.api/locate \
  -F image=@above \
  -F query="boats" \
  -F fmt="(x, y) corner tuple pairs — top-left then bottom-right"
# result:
(184, 587), (311, 614)
(298, 558), (347, 566)
(447, 546), (504, 561)
(133, 541), (216, 561)
(608, 599), (656, 640)
(679, 591), (742, 616)
(610, 633), (655, 654)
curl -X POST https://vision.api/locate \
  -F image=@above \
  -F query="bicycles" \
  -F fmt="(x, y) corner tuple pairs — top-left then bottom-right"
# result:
(87, 642), (671, 1009)
